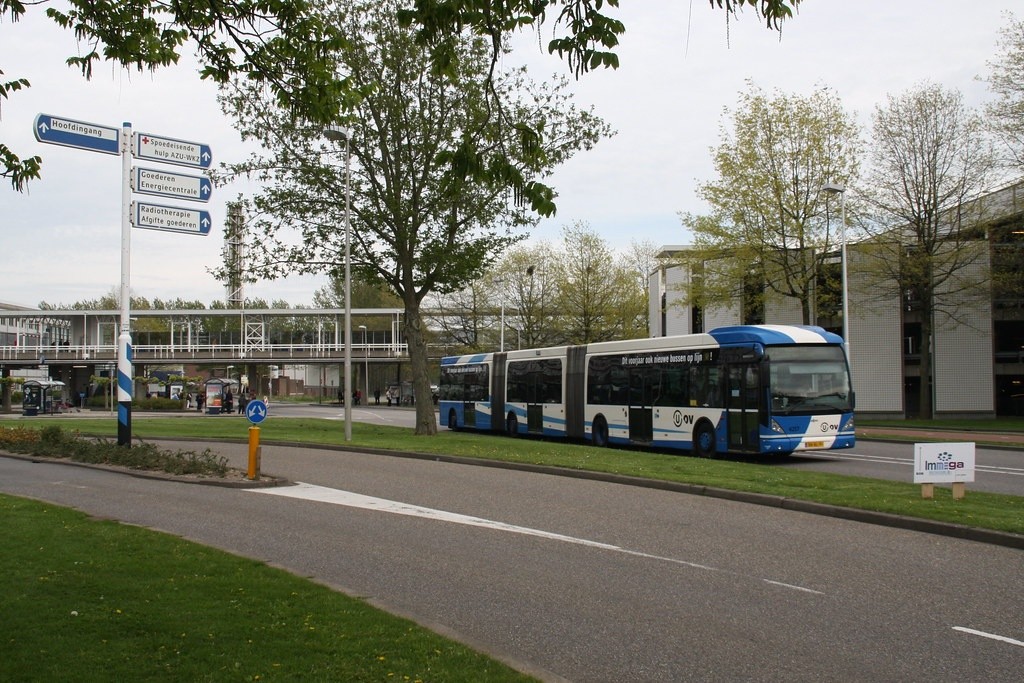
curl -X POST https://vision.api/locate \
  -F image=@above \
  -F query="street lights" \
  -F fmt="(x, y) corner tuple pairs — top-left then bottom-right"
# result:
(359, 325), (369, 405)
(824, 182), (849, 396)
(227, 366), (234, 380)
(322, 125), (354, 444)
(493, 278), (505, 351)
(181, 372), (185, 377)
(510, 306), (520, 350)
(108, 361), (115, 415)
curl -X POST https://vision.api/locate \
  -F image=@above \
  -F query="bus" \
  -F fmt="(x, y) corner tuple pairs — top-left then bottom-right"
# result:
(438, 324), (857, 460)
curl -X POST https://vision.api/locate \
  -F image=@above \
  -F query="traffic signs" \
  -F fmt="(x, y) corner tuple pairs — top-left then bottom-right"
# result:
(33, 113), (121, 156)
(132, 134), (213, 238)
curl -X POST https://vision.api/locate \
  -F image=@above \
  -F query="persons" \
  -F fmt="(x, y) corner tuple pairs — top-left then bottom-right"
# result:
(353, 388), (361, 406)
(51, 339), (70, 353)
(226, 389), (232, 414)
(250, 390), (256, 400)
(374, 388), (380, 405)
(145, 391), (160, 400)
(237, 393), (246, 414)
(174, 392), (180, 400)
(338, 390), (342, 404)
(195, 390), (204, 410)
(394, 386), (400, 406)
(386, 388), (393, 406)
(186, 391), (192, 409)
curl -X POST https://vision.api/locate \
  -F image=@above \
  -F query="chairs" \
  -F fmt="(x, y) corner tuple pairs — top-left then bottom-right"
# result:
(702, 379), (715, 404)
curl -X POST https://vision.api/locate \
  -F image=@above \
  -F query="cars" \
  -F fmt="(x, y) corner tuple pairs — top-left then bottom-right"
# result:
(389, 387), (398, 397)
(431, 385), (439, 396)
